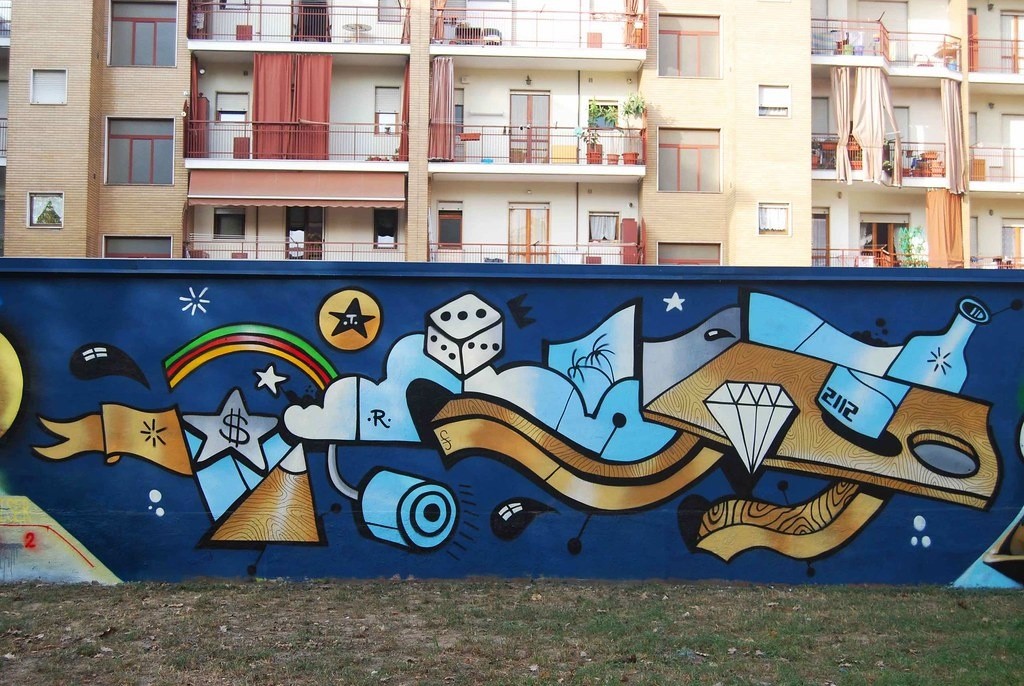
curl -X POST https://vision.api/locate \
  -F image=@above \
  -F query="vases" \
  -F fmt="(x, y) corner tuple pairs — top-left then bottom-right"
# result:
(902, 150), (931, 177)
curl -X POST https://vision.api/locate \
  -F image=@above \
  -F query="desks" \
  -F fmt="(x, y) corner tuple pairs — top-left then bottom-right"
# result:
(456, 27), (481, 45)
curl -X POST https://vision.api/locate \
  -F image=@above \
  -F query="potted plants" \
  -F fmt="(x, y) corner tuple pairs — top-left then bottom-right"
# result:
(581, 97), (648, 166)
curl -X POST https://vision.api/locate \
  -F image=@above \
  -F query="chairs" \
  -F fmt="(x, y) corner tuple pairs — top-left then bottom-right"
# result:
(481, 28), (503, 45)
